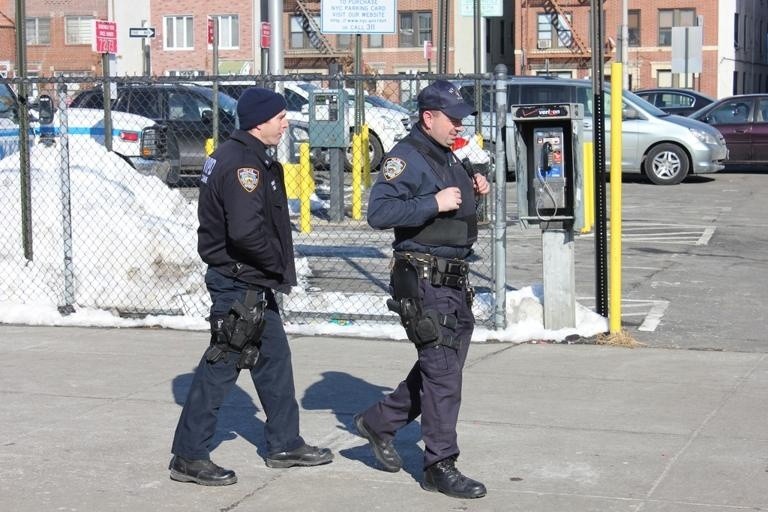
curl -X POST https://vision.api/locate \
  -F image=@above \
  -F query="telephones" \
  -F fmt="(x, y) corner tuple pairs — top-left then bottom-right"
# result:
(543, 142), (552, 171)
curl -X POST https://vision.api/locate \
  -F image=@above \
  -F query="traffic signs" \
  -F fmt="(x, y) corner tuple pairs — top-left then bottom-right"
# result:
(129, 27), (155, 38)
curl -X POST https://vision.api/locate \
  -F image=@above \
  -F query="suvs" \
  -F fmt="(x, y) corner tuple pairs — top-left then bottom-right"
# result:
(0, 72), (172, 190)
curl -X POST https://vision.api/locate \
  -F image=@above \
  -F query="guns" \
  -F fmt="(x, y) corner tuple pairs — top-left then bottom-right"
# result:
(387, 299), (418, 325)
(231, 299), (262, 348)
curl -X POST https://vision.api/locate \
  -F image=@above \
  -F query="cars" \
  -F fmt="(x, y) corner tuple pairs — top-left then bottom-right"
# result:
(684, 92), (766, 167)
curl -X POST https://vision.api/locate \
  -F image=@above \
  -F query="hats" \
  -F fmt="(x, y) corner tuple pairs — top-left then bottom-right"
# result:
(419, 79), (481, 119)
(237, 87), (288, 126)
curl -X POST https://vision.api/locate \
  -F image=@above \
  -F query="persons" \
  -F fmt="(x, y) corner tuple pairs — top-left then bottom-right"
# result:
(353, 80), (490, 499)
(170, 88), (335, 486)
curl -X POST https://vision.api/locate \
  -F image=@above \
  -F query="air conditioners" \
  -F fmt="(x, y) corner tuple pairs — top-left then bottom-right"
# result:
(536, 39), (550, 49)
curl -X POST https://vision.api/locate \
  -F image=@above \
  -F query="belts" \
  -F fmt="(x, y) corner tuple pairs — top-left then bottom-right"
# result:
(393, 249), (468, 287)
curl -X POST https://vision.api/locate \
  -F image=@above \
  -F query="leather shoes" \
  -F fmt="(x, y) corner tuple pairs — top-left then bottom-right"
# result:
(266, 444), (333, 466)
(422, 459), (486, 498)
(169, 455), (238, 485)
(355, 414), (403, 472)
